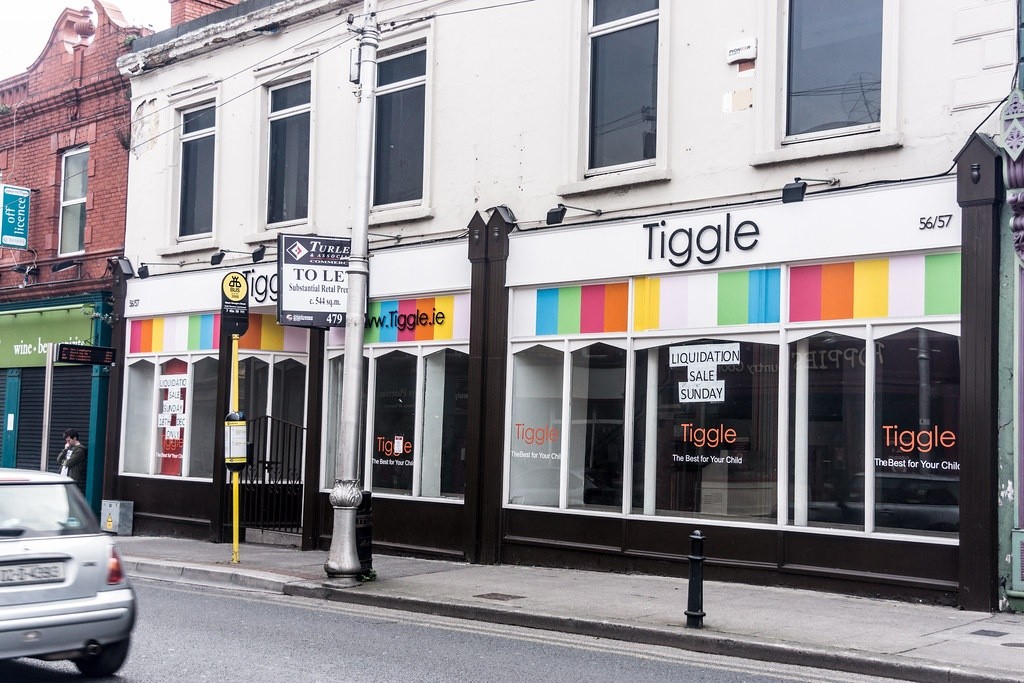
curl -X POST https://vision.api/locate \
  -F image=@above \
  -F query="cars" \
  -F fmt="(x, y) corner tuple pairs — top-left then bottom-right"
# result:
(509, 467), (597, 505)
(770, 473), (960, 533)
(0, 468), (137, 678)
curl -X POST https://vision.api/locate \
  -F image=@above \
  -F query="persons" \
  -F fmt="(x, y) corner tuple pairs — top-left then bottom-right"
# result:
(58, 430), (87, 481)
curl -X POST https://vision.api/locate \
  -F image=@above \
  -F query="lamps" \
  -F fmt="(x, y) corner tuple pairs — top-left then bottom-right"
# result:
(252, 244), (278, 264)
(210, 249), (253, 266)
(781, 177), (836, 203)
(53, 260), (83, 272)
(547, 203), (601, 224)
(115, 259), (133, 280)
(12, 266), (40, 277)
(138, 261), (185, 279)
